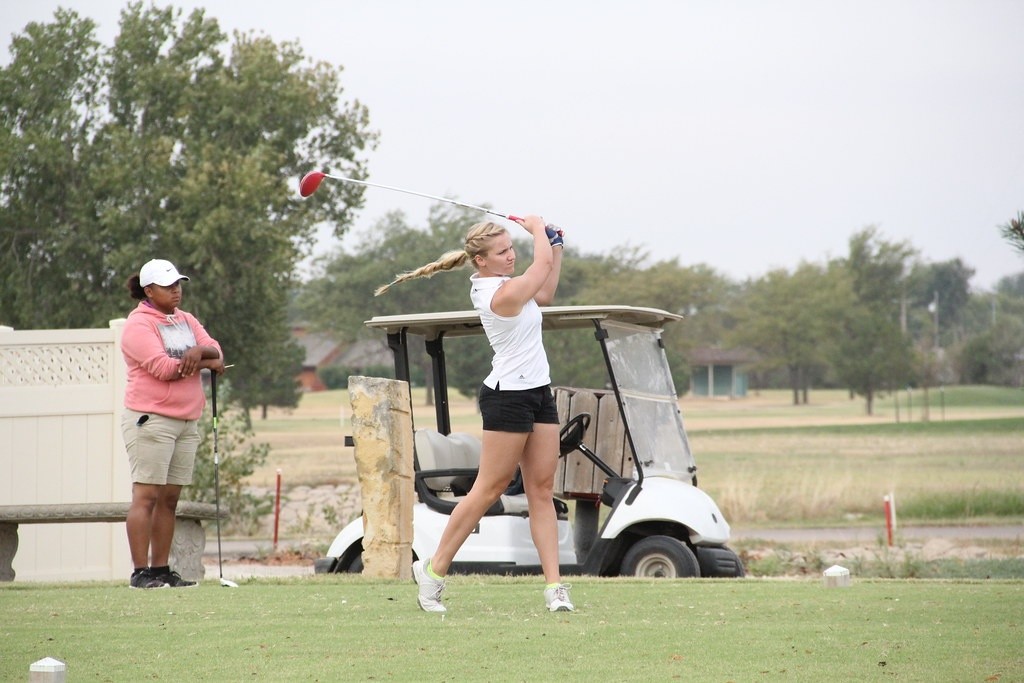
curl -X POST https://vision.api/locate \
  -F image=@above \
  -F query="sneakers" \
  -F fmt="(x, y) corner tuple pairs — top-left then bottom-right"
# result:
(129, 571), (169, 589)
(543, 585), (575, 613)
(412, 559), (446, 611)
(150, 567), (198, 589)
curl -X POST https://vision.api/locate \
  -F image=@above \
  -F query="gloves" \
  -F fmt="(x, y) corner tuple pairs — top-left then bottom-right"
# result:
(545, 224), (563, 250)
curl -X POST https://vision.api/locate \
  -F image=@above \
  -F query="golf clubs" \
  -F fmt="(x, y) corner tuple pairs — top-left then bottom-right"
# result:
(209, 367), (240, 588)
(297, 170), (566, 239)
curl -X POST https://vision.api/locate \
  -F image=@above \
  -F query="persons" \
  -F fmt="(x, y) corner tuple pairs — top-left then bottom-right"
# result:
(375, 215), (575, 613)
(121, 259), (224, 589)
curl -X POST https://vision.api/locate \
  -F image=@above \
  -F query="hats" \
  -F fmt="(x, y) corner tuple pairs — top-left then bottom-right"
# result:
(139, 258), (190, 288)
